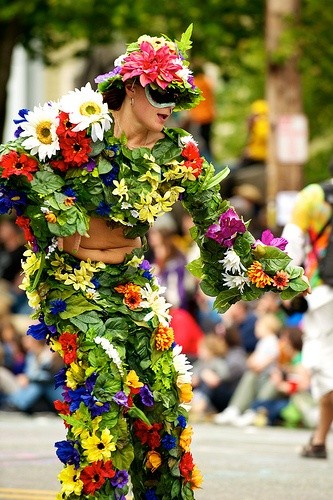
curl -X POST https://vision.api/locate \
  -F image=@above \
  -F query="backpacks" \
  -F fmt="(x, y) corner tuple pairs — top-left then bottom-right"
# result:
(310, 180), (333, 290)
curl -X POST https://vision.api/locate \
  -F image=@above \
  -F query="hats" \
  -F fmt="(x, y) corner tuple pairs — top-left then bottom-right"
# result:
(95, 24), (204, 109)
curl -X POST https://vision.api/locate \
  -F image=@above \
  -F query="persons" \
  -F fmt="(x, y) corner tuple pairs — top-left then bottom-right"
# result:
(0, 176), (333, 459)
(0, 24), (312, 500)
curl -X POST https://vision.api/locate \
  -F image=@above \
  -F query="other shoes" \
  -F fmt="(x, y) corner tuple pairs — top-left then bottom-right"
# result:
(301, 437), (326, 458)
(235, 409), (256, 428)
(214, 407), (243, 425)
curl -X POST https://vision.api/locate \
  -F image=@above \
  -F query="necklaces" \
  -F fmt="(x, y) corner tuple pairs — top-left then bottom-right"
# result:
(112, 109), (158, 148)
